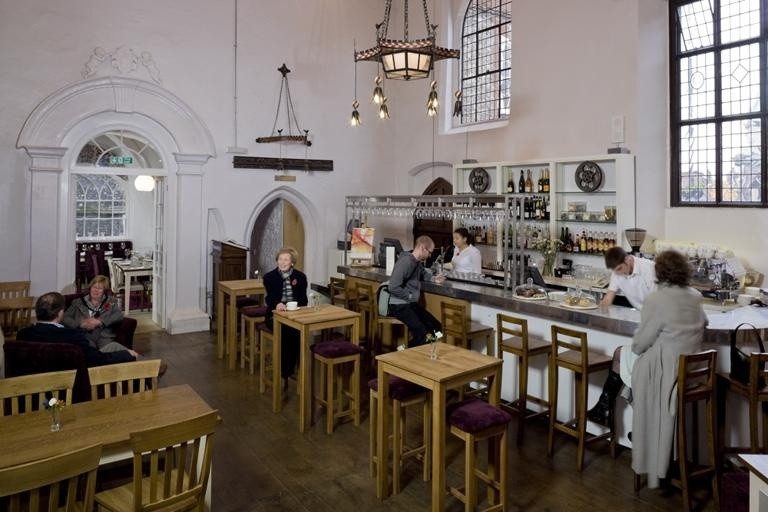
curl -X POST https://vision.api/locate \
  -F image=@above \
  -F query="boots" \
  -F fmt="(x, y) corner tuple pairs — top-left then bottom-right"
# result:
(587, 370), (624, 427)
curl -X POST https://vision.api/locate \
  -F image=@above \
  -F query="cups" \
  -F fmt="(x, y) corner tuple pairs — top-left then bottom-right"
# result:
(560, 201), (615, 221)
(286, 302), (298, 308)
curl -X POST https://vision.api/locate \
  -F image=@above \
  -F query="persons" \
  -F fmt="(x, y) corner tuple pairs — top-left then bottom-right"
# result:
(585, 250), (708, 491)
(263, 247), (309, 378)
(60, 275), (123, 348)
(386, 236), (446, 348)
(17, 291), (167, 390)
(450, 227), (482, 276)
(598, 247), (659, 311)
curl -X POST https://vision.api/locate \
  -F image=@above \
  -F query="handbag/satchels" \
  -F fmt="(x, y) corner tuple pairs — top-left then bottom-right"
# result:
(377, 280), (391, 316)
(728, 322), (767, 386)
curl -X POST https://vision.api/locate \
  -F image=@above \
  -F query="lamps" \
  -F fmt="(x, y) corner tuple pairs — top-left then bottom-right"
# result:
(348, 0), (464, 128)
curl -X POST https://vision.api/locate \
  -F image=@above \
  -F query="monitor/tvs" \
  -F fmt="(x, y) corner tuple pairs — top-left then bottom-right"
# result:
(383, 237), (404, 255)
(515, 267), (547, 289)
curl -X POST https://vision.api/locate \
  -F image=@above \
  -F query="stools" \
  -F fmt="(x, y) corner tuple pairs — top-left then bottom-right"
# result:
(226, 277), (381, 435)
(368, 277), (768, 512)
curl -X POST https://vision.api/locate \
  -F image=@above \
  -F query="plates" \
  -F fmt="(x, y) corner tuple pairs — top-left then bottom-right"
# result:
(287, 308), (301, 310)
(511, 293), (549, 302)
(561, 301), (599, 310)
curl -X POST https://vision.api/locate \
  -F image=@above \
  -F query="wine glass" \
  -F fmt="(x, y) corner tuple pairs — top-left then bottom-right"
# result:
(572, 264), (608, 290)
(440, 268), (489, 283)
(349, 203), (506, 222)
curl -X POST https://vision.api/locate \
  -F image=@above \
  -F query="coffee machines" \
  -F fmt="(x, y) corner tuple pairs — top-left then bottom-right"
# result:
(625, 229), (648, 257)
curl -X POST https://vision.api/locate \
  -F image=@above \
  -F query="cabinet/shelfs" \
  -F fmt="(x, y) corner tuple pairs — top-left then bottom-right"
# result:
(451, 153), (637, 257)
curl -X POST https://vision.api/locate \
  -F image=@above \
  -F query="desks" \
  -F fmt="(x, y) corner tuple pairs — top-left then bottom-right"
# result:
(211, 240), (248, 334)
(217, 279), (266, 370)
(112, 261), (153, 315)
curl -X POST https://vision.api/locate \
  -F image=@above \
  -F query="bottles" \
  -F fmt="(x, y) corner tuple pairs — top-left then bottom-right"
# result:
(468, 222), (542, 248)
(507, 169), (551, 218)
(559, 225), (618, 255)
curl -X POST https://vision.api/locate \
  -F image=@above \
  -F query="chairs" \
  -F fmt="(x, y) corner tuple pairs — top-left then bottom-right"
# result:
(2, 281), (224, 511)
